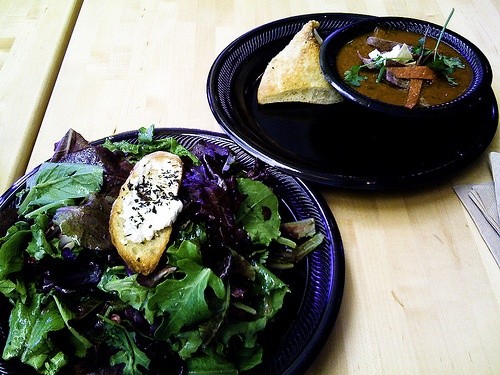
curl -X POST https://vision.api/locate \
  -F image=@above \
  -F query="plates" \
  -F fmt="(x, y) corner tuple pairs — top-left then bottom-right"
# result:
(0, 126), (344, 375)
(206, 13), (498, 196)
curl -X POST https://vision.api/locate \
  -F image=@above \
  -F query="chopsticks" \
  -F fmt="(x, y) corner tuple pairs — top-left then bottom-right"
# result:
(4, 1), (88, 189)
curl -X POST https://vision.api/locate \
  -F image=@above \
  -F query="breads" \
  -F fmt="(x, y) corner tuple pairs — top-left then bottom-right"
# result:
(256, 19), (343, 104)
(109, 150), (183, 274)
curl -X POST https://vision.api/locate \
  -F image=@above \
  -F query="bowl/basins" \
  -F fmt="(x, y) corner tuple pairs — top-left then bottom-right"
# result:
(319, 16), (483, 118)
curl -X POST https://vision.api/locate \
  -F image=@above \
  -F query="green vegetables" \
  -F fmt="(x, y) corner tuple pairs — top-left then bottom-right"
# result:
(0, 124), (326, 375)
(344, 6), (467, 88)
